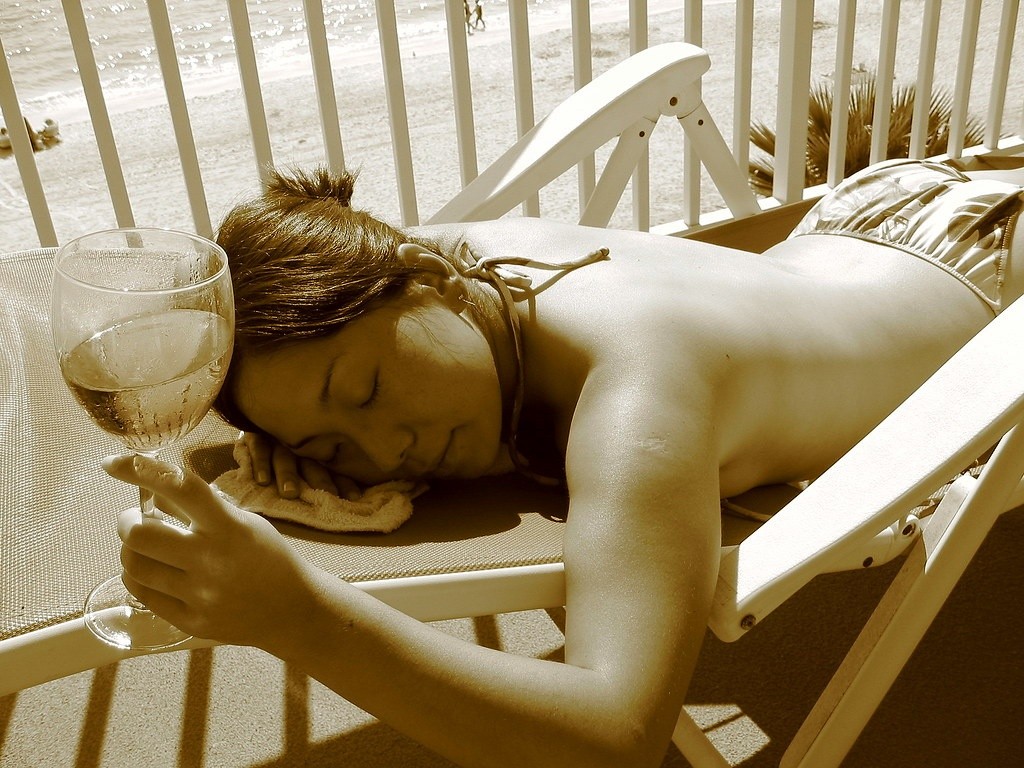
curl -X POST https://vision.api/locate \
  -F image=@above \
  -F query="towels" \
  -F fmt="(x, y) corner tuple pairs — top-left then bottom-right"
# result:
(209, 432), (429, 533)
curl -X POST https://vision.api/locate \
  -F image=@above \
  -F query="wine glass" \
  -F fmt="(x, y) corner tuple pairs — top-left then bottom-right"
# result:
(49, 228), (236, 652)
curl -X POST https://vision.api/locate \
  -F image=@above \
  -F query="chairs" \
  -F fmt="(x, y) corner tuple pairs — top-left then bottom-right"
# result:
(0, 42), (1024, 768)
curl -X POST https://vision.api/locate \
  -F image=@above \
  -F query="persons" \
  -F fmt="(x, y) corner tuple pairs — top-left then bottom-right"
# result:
(0, 119), (54, 140)
(102, 157), (1023, 768)
(464, 0), (485, 33)
